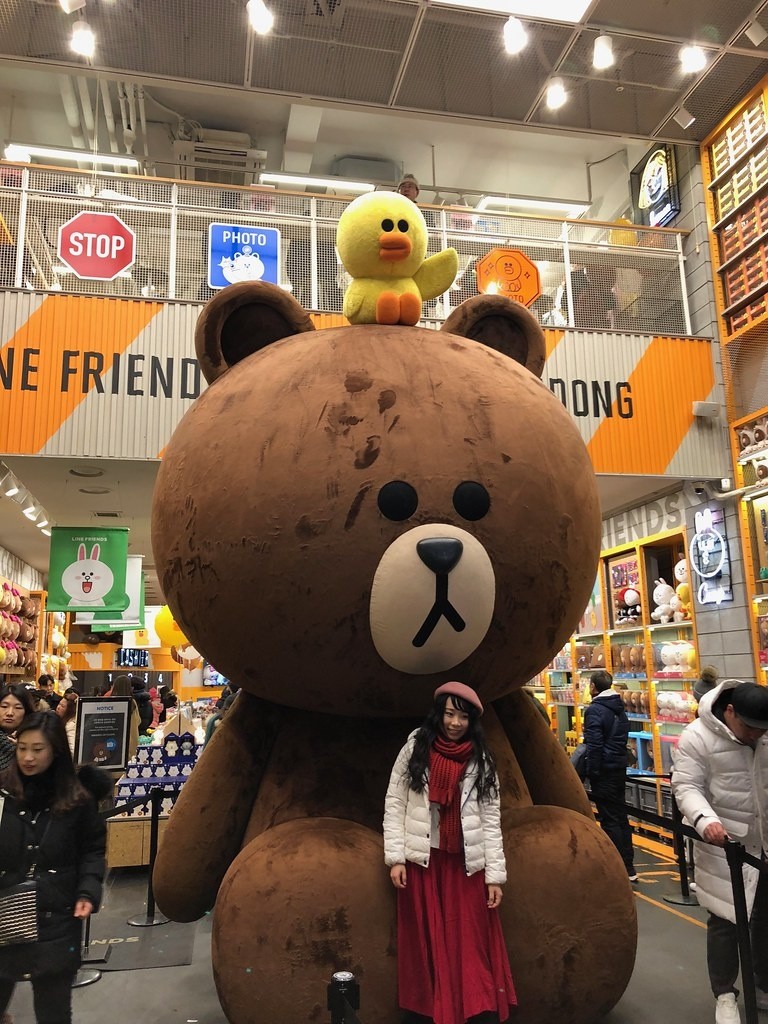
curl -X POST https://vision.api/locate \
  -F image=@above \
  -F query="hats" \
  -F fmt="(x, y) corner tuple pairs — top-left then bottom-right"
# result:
(19, 683), (45, 696)
(693, 665), (718, 700)
(149, 687), (158, 698)
(434, 682), (483, 719)
(732, 682), (768, 729)
(160, 686), (170, 694)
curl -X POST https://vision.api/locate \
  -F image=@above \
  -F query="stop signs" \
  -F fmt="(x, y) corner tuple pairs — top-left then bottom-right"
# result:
(57, 210), (137, 282)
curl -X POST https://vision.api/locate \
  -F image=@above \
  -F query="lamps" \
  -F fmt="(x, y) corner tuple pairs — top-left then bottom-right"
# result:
(502, 15), (529, 56)
(10, 485), (29, 505)
(673, 102), (696, 130)
(471, 198), (488, 225)
(483, 193), (595, 210)
(3, 474), (19, 497)
(0, 464), (9, 485)
(677, 42), (707, 74)
(24, 503), (43, 521)
(246, 0), (274, 37)
(544, 75), (568, 112)
(22, 496), (36, 514)
(5, 138), (142, 171)
(34, 512), (49, 528)
(592, 31), (615, 71)
(66, 15), (98, 60)
(39, 520), (57, 536)
(255, 169), (380, 194)
(57, 0), (86, 14)
(744, 20), (768, 47)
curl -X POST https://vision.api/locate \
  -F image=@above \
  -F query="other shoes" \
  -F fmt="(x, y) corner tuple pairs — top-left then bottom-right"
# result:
(627, 869), (638, 882)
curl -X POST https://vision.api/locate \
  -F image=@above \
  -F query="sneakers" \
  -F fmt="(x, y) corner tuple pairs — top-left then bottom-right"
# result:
(756, 990), (768, 1009)
(715, 992), (741, 1024)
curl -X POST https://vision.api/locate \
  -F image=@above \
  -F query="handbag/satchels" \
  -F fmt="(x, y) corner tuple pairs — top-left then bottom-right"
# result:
(570, 743), (588, 783)
(0, 879), (39, 945)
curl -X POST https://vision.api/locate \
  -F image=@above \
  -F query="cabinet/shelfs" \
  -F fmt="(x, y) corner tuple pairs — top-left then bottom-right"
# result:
(519, 528), (701, 839)
(700, 74), (768, 686)
(0, 576), (71, 695)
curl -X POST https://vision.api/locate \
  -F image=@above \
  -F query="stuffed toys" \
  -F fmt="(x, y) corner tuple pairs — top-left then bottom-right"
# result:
(615, 559), (698, 720)
(150, 281), (637, 1024)
(0, 580), (40, 668)
(337, 191), (458, 326)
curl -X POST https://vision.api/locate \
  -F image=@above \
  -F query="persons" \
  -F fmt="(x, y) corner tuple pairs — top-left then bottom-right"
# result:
(383, 682), (517, 1024)
(0, 711), (117, 1024)
(397, 174), (435, 227)
(671, 678), (768, 1024)
(690, 664), (718, 891)
(198, 192), (244, 300)
(584, 670), (639, 881)
(18, 675), (177, 781)
(0, 683), (36, 775)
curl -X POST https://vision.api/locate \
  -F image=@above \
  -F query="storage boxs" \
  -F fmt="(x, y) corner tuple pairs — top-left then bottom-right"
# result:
(111, 731), (203, 817)
